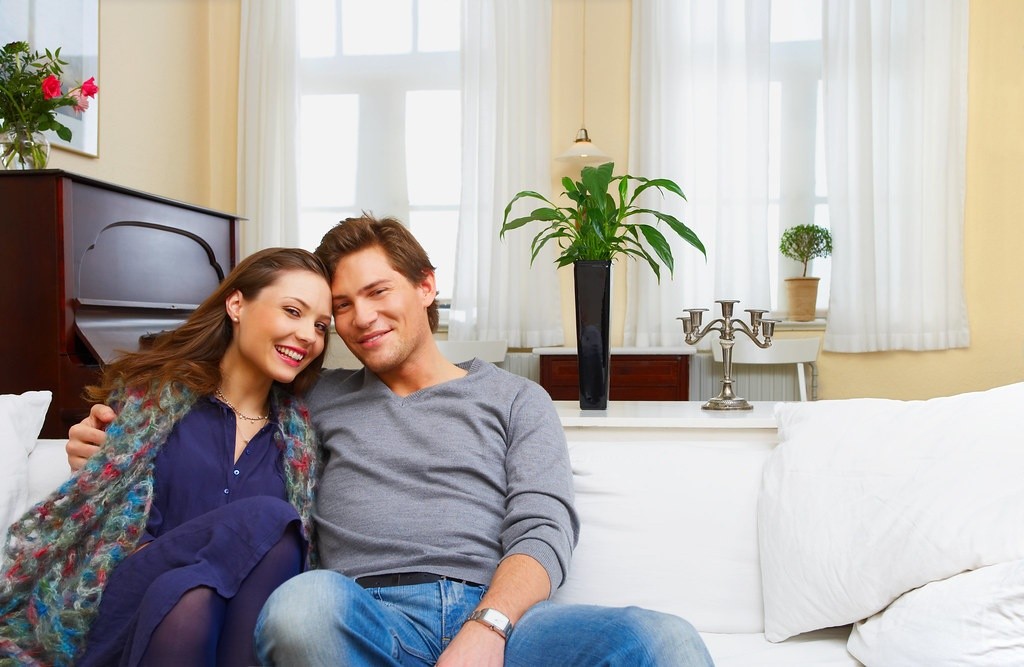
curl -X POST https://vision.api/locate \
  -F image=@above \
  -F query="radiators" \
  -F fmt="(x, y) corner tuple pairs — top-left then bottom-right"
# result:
(498, 352), (818, 400)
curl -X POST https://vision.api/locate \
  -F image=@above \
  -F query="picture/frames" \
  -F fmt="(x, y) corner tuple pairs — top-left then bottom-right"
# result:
(0, 0), (101, 158)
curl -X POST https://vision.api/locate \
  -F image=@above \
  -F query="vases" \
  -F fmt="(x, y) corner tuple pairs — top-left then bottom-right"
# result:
(778, 224), (833, 322)
(0, 122), (50, 170)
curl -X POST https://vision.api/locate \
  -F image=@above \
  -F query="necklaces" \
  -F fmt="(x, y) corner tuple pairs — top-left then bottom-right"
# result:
(213, 386), (272, 425)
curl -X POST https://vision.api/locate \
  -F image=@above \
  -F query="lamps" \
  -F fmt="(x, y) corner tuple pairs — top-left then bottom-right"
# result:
(551, 0), (612, 161)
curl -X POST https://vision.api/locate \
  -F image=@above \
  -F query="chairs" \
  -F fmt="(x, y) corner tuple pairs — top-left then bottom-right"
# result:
(435, 339), (510, 367)
(711, 338), (821, 401)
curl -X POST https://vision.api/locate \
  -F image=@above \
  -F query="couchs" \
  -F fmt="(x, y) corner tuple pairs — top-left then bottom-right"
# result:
(26, 438), (857, 667)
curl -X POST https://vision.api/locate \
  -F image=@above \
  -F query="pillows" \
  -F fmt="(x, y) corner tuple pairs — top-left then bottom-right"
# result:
(760, 381), (1024, 644)
(551, 438), (763, 632)
(0, 388), (53, 565)
(845, 560), (1023, 667)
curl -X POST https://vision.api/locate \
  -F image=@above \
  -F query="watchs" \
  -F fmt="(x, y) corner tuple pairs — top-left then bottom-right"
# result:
(458, 608), (514, 644)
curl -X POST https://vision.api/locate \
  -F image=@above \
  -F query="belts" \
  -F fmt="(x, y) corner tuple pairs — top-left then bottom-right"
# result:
(355, 570), (483, 589)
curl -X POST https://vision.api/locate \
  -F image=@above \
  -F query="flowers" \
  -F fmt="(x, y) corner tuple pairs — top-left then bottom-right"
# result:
(0, 40), (99, 169)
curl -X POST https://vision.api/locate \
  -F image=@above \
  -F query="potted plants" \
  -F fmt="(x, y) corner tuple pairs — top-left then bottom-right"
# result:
(498, 161), (707, 410)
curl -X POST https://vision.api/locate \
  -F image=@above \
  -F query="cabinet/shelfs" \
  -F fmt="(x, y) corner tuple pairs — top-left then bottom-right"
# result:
(531, 346), (697, 401)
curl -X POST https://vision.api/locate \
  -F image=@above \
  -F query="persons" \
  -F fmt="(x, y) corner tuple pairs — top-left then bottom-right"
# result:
(55, 246), (335, 667)
(65, 211), (716, 667)
